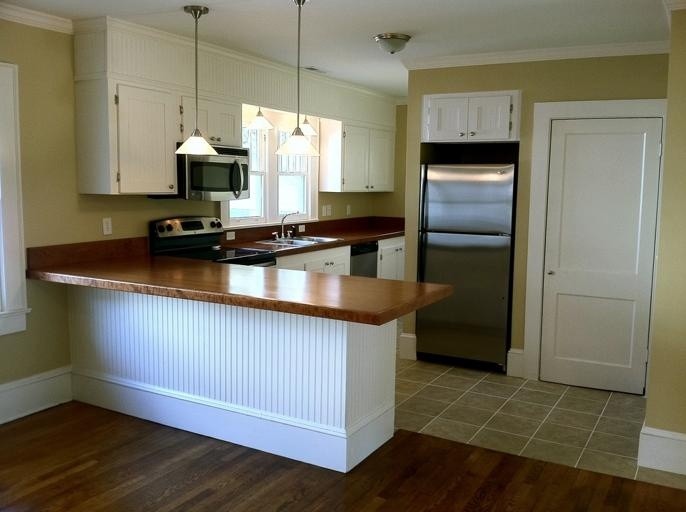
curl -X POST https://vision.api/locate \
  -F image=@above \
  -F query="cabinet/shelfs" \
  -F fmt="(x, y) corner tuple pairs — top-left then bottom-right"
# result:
(178, 91), (242, 148)
(377, 235), (404, 282)
(319, 117), (398, 193)
(421, 88), (522, 143)
(305, 245), (350, 281)
(72, 77), (180, 195)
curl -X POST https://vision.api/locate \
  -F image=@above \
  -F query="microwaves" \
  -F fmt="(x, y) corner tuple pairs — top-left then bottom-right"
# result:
(184, 154), (250, 202)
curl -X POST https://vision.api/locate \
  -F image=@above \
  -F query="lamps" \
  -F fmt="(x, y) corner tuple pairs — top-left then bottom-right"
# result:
(373, 33), (411, 57)
(246, 106), (273, 130)
(174, 6), (221, 155)
(276, 0), (321, 159)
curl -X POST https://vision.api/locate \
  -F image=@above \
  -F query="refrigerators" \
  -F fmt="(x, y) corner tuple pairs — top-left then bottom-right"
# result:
(416, 163), (516, 375)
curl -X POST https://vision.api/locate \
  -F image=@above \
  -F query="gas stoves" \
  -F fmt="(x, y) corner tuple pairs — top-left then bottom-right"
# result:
(170, 243), (270, 262)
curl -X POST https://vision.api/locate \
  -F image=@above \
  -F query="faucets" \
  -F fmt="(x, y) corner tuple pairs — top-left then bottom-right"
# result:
(282, 212), (299, 238)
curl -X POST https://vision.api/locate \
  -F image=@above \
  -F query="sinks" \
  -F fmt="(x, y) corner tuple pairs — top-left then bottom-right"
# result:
(255, 239), (316, 247)
(294, 236), (343, 243)
